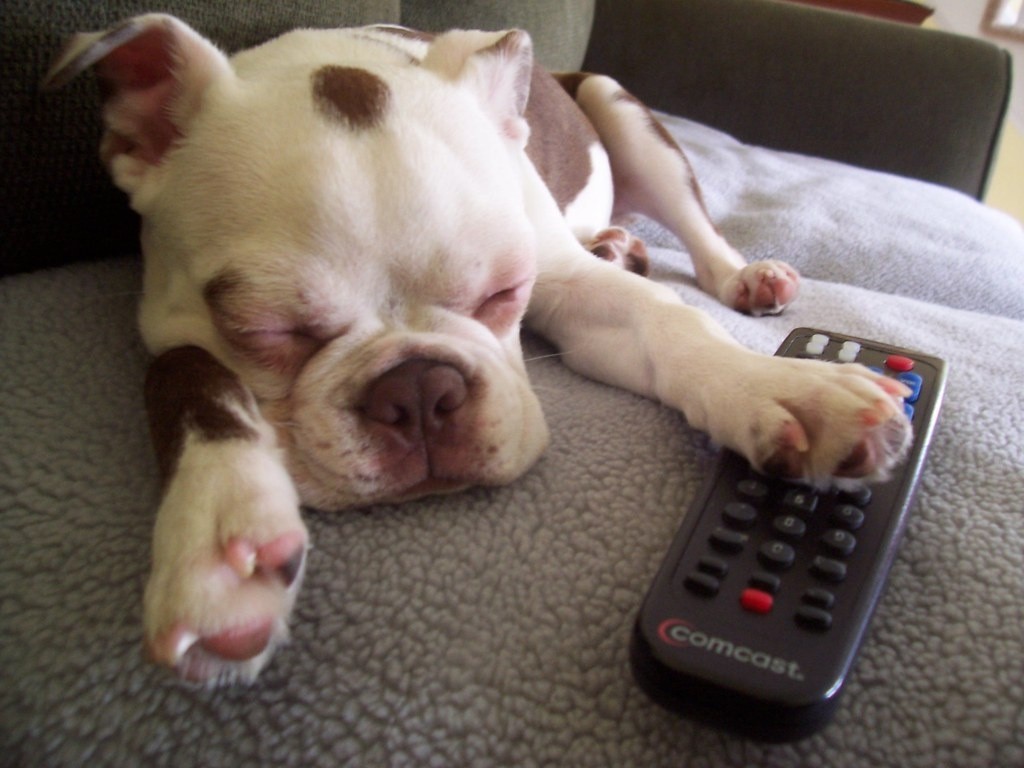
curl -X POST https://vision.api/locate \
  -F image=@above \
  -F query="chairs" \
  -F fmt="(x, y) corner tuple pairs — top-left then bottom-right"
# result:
(578, 0), (1015, 202)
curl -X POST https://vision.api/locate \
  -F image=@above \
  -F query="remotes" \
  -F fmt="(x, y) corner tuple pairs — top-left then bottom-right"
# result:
(629, 327), (950, 745)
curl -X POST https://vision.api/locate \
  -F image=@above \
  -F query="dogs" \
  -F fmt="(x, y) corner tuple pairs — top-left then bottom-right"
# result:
(37, 11), (914, 690)
